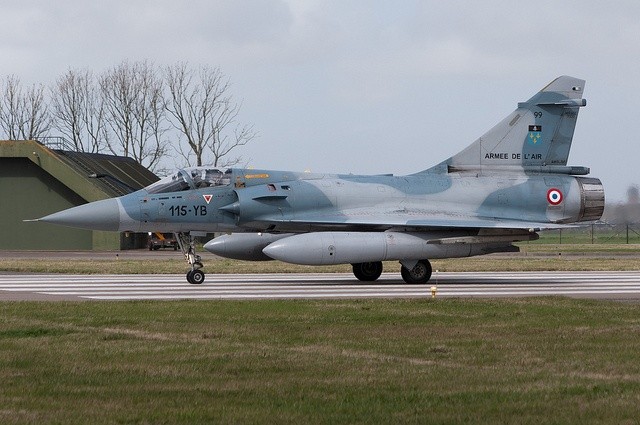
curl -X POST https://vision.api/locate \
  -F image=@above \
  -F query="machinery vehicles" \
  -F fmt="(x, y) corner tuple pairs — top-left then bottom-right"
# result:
(147, 232), (180, 251)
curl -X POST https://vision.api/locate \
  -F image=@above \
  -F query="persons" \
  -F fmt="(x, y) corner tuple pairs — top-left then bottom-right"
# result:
(190, 170), (207, 190)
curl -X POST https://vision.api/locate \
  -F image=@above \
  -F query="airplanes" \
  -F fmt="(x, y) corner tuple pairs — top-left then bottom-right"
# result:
(23, 75), (605, 283)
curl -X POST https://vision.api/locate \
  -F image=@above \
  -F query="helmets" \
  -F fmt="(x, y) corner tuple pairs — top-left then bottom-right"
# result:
(191, 169), (202, 179)
